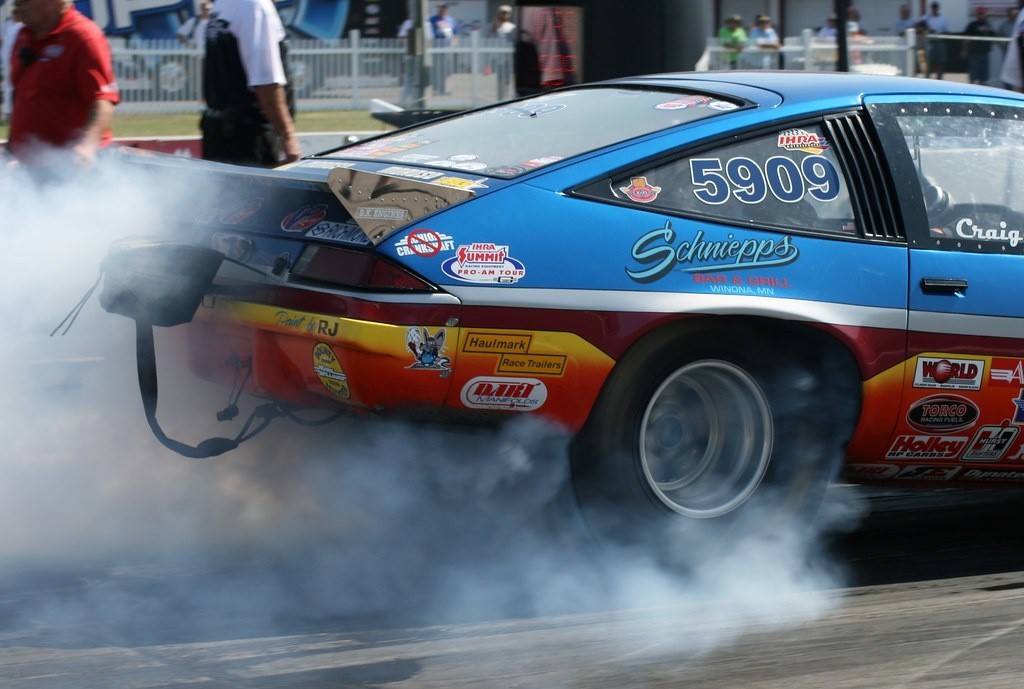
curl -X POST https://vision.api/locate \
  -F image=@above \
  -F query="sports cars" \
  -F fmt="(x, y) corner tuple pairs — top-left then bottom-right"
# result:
(80, 64), (1024, 574)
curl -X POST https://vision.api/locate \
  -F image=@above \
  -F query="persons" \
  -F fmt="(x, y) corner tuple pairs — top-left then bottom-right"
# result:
(482, 4), (518, 102)
(429, 5), (459, 95)
(717, 14), (782, 70)
(817, 9), (862, 68)
(960, 7), (995, 85)
(0, 0), (121, 166)
(176, 0), (301, 169)
(397, 17), (434, 88)
(919, 2), (949, 80)
(998, 0), (1024, 95)
(894, 4), (921, 74)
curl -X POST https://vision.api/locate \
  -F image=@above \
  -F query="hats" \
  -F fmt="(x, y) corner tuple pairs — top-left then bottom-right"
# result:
(976, 6), (988, 13)
(496, 5), (512, 13)
(756, 16), (771, 25)
(723, 14), (743, 23)
(436, 2), (449, 7)
